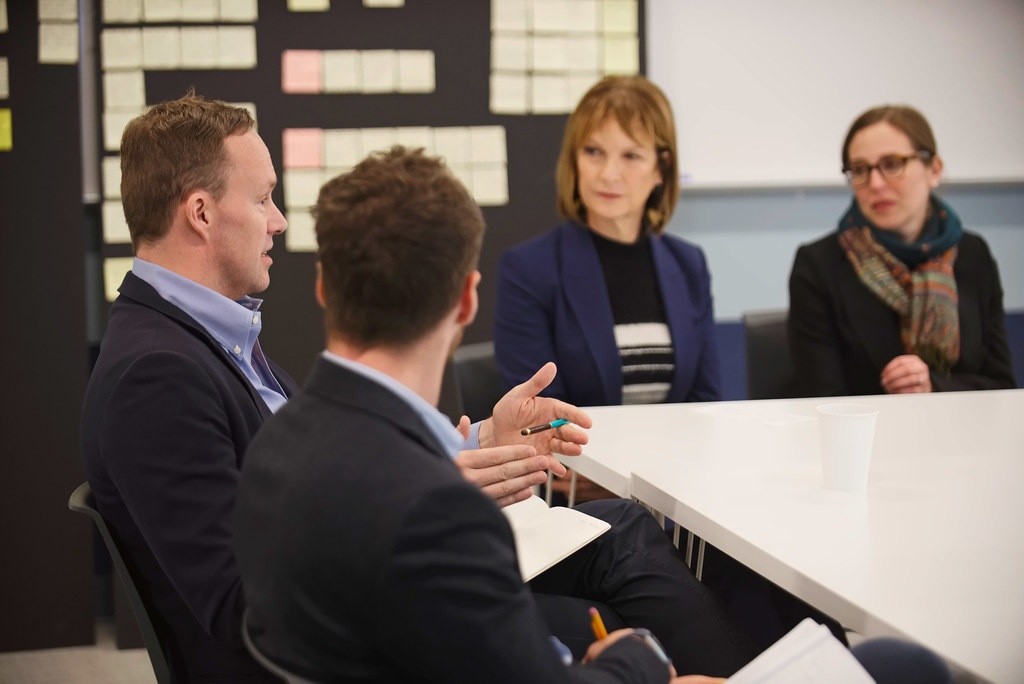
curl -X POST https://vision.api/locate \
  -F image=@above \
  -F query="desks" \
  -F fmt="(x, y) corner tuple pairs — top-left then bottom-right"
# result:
(550, 389), (1024, 684)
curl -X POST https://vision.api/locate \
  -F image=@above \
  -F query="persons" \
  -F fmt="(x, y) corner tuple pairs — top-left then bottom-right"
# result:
(233, 144), (956, 681)
(490, 73), (724, 403)
(782, 105), (1012, 397)
(72, 96), (738, 683)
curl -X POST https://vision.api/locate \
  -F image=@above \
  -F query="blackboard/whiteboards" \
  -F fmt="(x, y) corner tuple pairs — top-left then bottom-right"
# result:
(645, 0), (1024, 199)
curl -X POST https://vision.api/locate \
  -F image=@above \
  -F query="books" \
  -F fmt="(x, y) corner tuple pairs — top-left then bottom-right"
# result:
(499, 494), (613, 585)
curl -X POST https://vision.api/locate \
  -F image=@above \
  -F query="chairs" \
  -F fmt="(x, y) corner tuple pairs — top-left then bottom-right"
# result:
(452, 340), (506, 425)
(744, 309), (799, 400)
(68, 482), (191, 684)
(240, 606), (316, 684)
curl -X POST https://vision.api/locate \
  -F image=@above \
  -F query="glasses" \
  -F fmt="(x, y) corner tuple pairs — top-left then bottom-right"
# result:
(842, 151), (931, 184)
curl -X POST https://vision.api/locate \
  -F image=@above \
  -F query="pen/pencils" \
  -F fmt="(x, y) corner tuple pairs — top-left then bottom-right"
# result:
(589, 607), (608, 640)
(521, 418), (570, 436)
